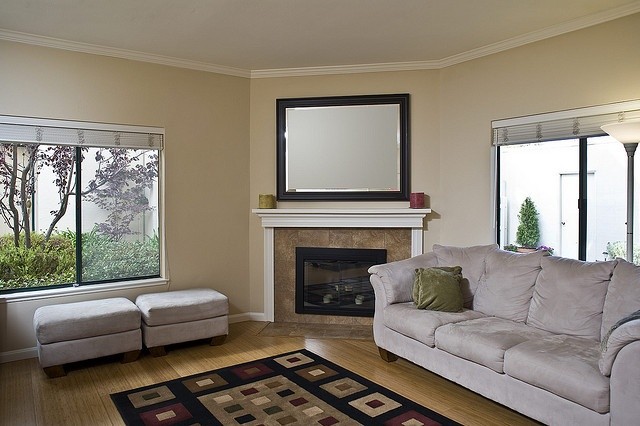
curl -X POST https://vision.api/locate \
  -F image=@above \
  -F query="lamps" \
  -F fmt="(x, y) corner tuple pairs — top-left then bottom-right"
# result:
(600, 118), (640, 262)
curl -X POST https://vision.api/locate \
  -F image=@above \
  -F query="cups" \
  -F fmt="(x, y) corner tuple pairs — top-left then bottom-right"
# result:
(259, 193), (274, 208)
(409, 191), (425, 209)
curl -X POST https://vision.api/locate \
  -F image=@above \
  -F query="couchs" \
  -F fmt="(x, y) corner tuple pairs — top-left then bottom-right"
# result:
(367, 244), (639, 426)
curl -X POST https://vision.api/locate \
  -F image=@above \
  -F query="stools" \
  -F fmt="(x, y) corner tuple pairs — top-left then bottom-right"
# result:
(33, 297), (142, 377)
(136, 286), (230, 357)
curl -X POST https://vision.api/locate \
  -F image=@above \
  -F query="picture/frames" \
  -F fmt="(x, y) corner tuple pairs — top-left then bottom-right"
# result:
(276, 92), (409, 201)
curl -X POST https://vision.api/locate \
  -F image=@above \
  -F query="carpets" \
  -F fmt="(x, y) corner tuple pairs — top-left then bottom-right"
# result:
(108, 348), (463, 425)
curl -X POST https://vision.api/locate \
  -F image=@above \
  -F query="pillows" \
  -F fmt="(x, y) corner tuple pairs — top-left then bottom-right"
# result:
(600, 257), (640, 345)
(412, 266), (463, 306)
(417, 268), (464, 313)
(472, 249), (548, 323)
(433, 242), (499, 306)
(527, 255), (618, 342)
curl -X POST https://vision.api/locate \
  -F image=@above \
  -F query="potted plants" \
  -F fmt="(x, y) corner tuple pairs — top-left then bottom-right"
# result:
(516, 197), (541, 253)
